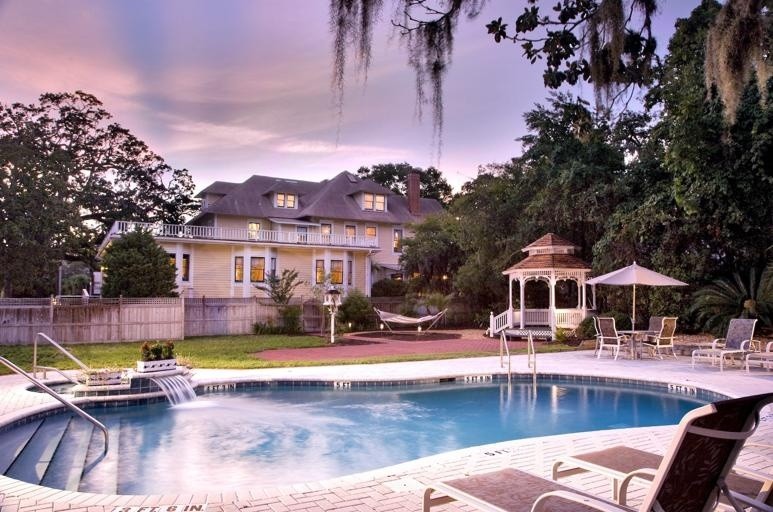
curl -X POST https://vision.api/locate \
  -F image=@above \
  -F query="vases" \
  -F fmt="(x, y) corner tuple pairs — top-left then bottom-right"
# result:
(136, 359), (178, 372)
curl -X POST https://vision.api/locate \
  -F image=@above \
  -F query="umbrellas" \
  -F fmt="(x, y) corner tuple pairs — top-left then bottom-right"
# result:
(582, 260), (689, 330)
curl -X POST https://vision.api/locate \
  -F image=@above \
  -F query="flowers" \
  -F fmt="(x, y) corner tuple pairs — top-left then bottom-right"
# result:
(140, 338), (174, 359)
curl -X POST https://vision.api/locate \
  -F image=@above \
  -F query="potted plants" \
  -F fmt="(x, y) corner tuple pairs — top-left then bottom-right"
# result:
(85, 360), (121, 385)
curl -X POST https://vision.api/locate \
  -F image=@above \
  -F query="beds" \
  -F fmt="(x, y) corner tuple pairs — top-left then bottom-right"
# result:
(372, 306), (449, 334)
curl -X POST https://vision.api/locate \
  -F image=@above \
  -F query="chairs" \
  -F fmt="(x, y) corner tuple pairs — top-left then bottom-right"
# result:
(423, 391), (773, 511)
(591, 313), (773, 376)
